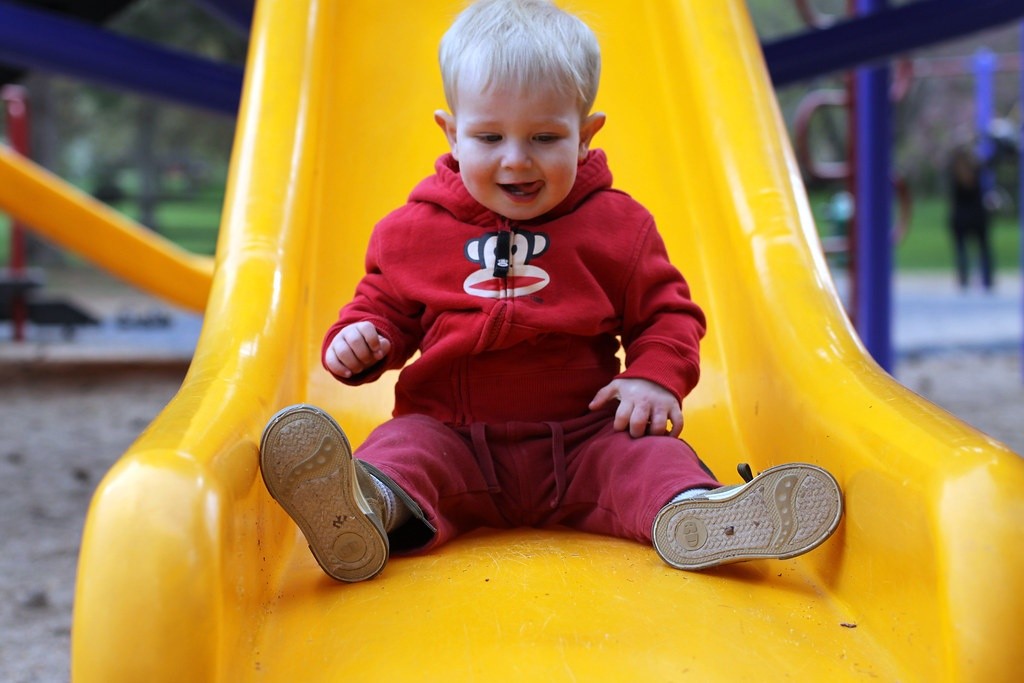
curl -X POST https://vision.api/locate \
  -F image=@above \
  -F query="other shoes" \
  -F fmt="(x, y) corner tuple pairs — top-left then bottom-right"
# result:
(652, 462), (844, 571)
(258, 404), (388, 582)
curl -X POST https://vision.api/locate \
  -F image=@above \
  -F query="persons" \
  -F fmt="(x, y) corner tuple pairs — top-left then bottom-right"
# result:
(259, 0), (843, 582)
(947, 147), (992, 291)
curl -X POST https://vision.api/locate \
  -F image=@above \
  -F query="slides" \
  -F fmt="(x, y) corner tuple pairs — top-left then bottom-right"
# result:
(71, 0), (1024, 683)
(0, 143), (216, 316)
